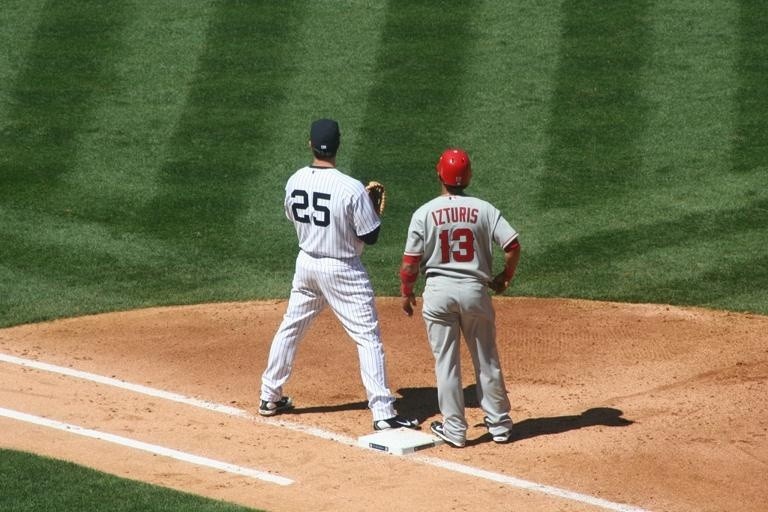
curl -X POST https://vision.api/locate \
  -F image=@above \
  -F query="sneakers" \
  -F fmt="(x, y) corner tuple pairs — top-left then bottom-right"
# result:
(431, 421), (466, 447)
(258, 396), (292, 416)
(374, 416), (419, 431)
(493, 430), (512, 442)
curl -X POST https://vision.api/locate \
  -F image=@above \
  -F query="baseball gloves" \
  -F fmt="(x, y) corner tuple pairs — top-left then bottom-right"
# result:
(364, 182), (385, 217)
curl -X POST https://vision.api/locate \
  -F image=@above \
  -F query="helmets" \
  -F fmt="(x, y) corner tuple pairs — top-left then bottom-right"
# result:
(436, 149), (471, 187)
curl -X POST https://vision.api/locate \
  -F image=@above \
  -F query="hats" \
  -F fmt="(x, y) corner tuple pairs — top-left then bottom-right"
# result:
(311, 119), (340, 150)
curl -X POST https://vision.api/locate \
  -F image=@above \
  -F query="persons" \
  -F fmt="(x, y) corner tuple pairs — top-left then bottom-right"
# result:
(256, 116), (422, 432)
(399, 148), (522, 449)
